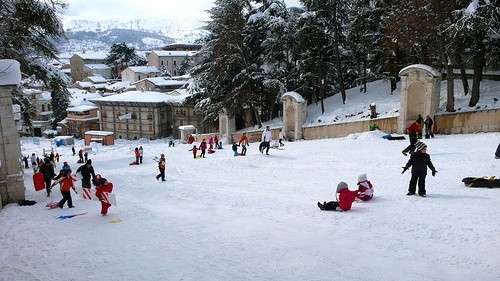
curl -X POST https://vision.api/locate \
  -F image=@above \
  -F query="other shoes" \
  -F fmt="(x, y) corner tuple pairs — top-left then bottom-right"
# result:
(418, 192), (426, 197)
(409, 151), (414, 154)
(324, 202), (326, 205)
(318, 202), (324, 210)
(402, 152), (407, 156)
(407, 192), (415, 195)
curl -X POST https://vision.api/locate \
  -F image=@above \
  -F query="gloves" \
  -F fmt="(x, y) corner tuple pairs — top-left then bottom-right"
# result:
(401, 167), (409, 173)
(431, 167), (437, 176)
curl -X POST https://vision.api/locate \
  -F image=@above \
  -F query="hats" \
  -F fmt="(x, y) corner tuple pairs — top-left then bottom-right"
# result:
(357, 174), (367, 182)
(414, 141), (427, 152)
(336, 181), (348, 193)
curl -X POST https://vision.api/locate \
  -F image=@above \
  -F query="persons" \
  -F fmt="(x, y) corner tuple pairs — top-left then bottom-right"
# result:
(233, 141), (240, 156)
(402, 118), (422, 156)
(416, 114), (424, 139)
(317, 181), (357, 211)
(402, 141), (437, 197)
(424, 115), (435, 139)
(495, 144), (500, 160)
(353, 173), (374, 201)
(259, 126), (272, 155)
(135, 146), (143, 164)
(239, 133), (249, 156)
(21, 147), (114, 216)
(156, 154), (166, 181)
(187, 134), (223, 158)
(370, 110), (378, 130)
(278, 128), (285, 146)
